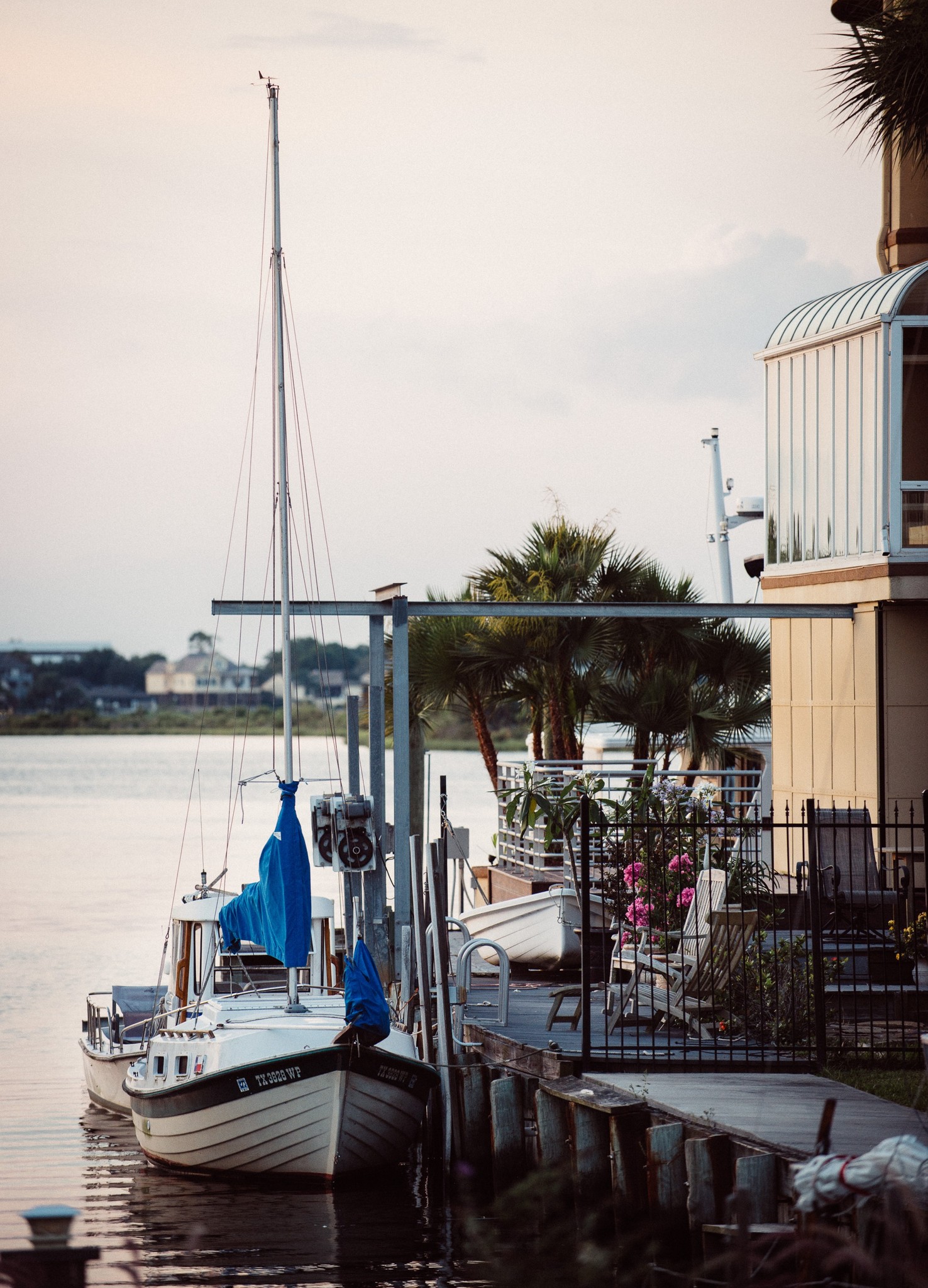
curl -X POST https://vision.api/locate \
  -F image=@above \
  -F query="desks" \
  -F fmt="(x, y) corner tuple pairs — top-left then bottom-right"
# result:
(873, 847), (924, 926)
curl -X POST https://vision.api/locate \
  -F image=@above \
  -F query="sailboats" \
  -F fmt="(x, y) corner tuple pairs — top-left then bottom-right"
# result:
(123, 71), (439, 1171)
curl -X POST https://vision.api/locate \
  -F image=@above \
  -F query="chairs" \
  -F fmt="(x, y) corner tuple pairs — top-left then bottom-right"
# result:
(603, 869), (731, 1016)
(795, 808), (910, 944)
(545, 909), (760, 1040)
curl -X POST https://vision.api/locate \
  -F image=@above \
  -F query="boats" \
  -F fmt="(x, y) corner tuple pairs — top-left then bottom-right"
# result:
(460, 884), (617, 969)
(78, 986), (167, 1118)
